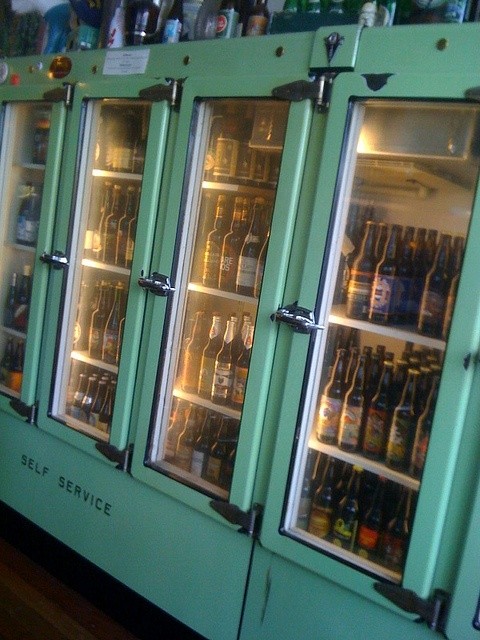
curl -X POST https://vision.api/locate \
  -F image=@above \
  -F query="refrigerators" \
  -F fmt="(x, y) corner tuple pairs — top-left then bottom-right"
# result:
(1, 20), (479, 639)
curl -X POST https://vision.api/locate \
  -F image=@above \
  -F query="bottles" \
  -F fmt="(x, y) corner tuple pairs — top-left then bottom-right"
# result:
(119, 114), (134, 172)
(70, 374), (86, 417)
(3, 273), (18, 327)
(378, 0), (397, 26)
(106, 0), (128, 47)
(198, 315), (222, 399)
(105, 280), (114, 319)
(408, 357), (420, 368)
(337, 353), (367, 452)
(129, 0), (161, 45)
(443, 274), (459, 340)
(166, 398), (183, 464)
(230, 315), (239, 353)
(378, 487), (412, 572)
(25, 181), (42, 246)
(191, 410), (212, 478)
(230, 324), (254, 409)
(363, 345), (373, 359)
(253, 147), (269, 188)
(358, 0), (378, 26)
(236, 198), (264, 296)
(223, 438), (237, 487)
(80, 376), (96, 422)
(308, 455), (336, 541)
(416, 366), (431, 415)
(385, 366), (421, 471)
(207, 415), (231, 484)
(368, 223), (404, 325)
(116, 317), (125, 366)
(32, 110), (50, 164)
(363, 360), (394, 461)
(369, 353), (383, 384)
(99, 385), (113, 432)
(236, 0), (250, 37)
(213, 100), (239, 183)
(89, 282), (107, 358)
(218, 197), (241, 292)
(374, 221), (390, 261)
(332, 460), (351, 503)
(296, 450), (313, 531)
(356, 207), (374, 250)
(101, 376), (109, 386)
(246, 0), (268, 35)
(240, 322), (251, 354)
(102, 283), (123, 364)
(161, 0), (184, 42)
(92, 373), (99, 386)
(93, 181), (112, 260)
(397, 225), (414, 321)
(238, 106), (256, 186)
(104, 185), (121, 264)
(133, 110), (148, 174)
(425, 227), (438, 266)
(115, 187), (136, 266)
(302, 0), (324, 13)
(346, 220), (377, 321)
(345, 347), (359, 386)
(0, 337), (13, 386)
(244, 316), (251, 326)
(402, 341), (414, 361)
(315, 348), (348, 445)
(418, 231), (452, 336)
(269, 149), (280, 190)
(333, 204), (360, 305)
(334, 327), (344, 355)
(384, 351), (395, 360)
(283, 0), (300, 14)
(9, 339), (24, 393)
(391, 359), (409, 402)
(217, 0), (240, 38)
(254, 225), (271, 298)
(329, 464), (364, 551)
(452, 232), (464, 275)
(310, 452), (326, 490)
(205, 109), (219, 182)
(181, 311), (206, 393)
(90, 381), (106, 427)
(111, 381), (117, 410)
(355, 474), (388, 559)
(126, 186), (141, 269)
(175, 404), (197, 471)
(203, 195), (225, 287)
(14, 264), (31, 332)
(431, 364), (442, 377)
(85, 280), (101, 348)
(416, 226), (427, 265)
(346, 328), (359, 351)
(409, 374), (440, 480)
(426, 355), (439, 368)
(241, 198), (249, 240)
(375, 345), (386, 356)
(16, 182), (32, 245)
(316, 325), (336, 413)
(327, 0), (346, 14)
(210, 320), (235, 406)
